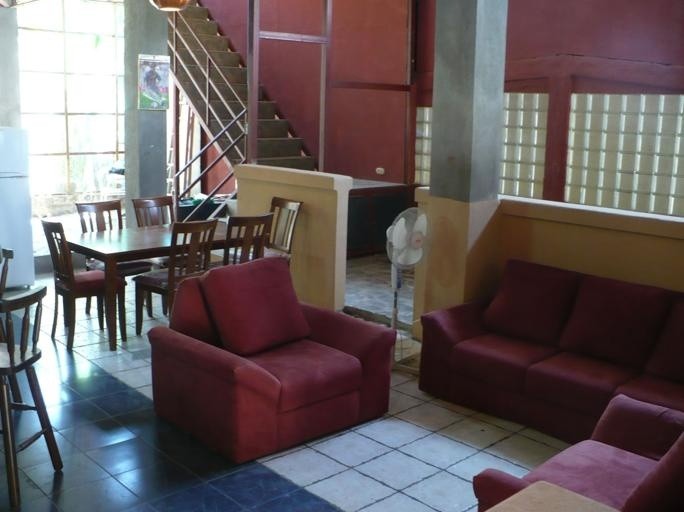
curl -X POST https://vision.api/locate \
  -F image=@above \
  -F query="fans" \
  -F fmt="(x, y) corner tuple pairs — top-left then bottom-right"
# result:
(378, 207), (432, 367)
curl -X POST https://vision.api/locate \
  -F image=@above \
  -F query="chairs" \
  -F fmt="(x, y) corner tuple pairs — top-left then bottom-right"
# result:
(209, 213), (274, 270)
(129, 196), (210, 267)
(239, 196), (303, 261)
(77, 201), (153, 335)
(133, 218), (219, 335)
(42, 220), (127, 351)
(0, 287), (64, 510)
(0, 247), (14, 343)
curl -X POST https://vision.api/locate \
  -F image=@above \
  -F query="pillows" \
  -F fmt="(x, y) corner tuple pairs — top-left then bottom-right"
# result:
(480, 258), (580, 347)
(200, 257), (312, 358)
(644, 292), (684, 384)
(557, 275), (674, 369)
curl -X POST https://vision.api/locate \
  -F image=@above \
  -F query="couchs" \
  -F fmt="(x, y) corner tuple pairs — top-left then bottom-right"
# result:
(462, 393), (682, 512)
(416, 258), (683, 448)
(148, 255), (397, 465)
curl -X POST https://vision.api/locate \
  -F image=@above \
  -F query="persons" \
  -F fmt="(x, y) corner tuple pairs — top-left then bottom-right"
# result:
(142, 62), (162, 88)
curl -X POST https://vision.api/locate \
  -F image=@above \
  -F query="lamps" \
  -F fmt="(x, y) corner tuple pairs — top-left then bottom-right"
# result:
(148, 0), (194, 12)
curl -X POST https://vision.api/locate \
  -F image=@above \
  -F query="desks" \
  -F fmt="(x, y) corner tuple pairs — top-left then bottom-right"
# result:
(55, 221), (270, 351)
(476, 481), (624, 512)
